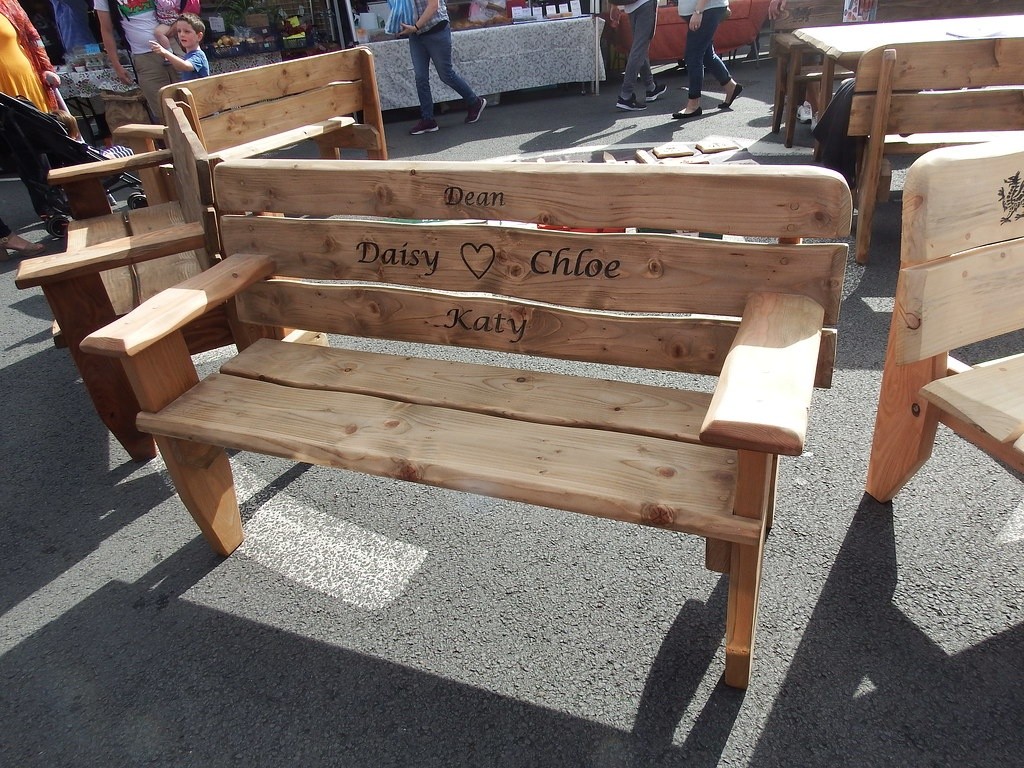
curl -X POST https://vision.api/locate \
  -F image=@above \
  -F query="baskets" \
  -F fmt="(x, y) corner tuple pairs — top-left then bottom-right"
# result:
(243, 40), (278, 54)
(210, 45), (240, 58)
(283, 34), (313, 49)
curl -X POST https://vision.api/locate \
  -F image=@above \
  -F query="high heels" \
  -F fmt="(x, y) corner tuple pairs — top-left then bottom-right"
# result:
(0, 231), (46, 262)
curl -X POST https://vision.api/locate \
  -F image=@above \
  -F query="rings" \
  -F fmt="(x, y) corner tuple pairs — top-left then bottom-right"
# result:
(695, 26), (697, 28)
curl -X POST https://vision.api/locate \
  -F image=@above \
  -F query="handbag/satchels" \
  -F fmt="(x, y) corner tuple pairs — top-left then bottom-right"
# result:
(384, 0), (415, 35)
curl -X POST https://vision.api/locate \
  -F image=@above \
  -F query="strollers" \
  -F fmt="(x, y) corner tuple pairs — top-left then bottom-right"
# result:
(0, 91), (149, 239)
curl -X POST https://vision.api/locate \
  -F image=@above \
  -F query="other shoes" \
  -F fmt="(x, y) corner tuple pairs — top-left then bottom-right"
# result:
(163, 51), (177, 66)
(796, 100), (812, 124)
(718, 82), (743, 108)
(672, 106), (702, 118)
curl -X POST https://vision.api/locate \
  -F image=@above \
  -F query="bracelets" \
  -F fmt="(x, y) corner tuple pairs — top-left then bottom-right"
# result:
(415, 24), (420, 31)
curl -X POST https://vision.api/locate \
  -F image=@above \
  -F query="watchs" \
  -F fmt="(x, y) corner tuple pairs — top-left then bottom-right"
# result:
(695, 10), (702, 15)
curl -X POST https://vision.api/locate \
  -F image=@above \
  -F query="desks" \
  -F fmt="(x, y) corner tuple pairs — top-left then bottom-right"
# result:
(355, 13), (607, 111)
(791, 14), (1024, 160)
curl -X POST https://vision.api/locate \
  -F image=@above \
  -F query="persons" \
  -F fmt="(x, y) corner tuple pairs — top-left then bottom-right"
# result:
(0, 0), (220, 261)
(399, 1), (487, 134)
(468, 0), (506, 23)
(610, 0), (822, 132)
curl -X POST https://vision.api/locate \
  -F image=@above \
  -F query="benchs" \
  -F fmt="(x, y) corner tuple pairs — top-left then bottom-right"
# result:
(847, 37), (1024, 263)
(15, 97), (226, 364)
(83, 157), (853, 689)
(864, 137), (1024, 503)
(112, 46), (389, 203)
(770, 0), (1024, 128)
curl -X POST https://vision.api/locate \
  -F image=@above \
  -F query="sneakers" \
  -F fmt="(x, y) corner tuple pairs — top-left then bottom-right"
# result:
(645, 81), (667, 102)
(409, 120), (439, 135)
(465, 97), (487, 123)
(615, 92), (647, 111)
